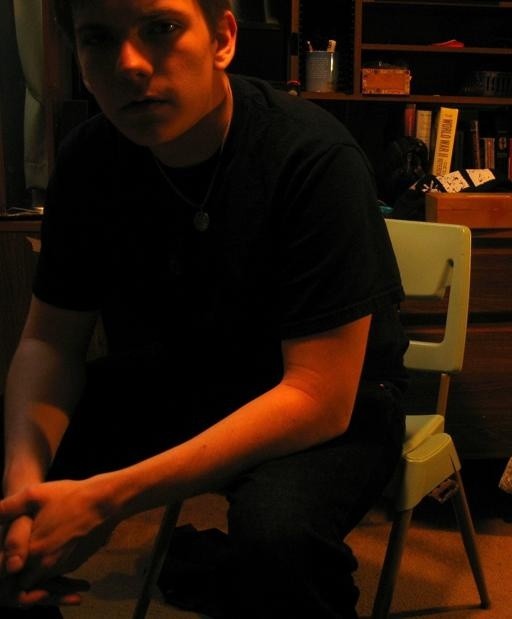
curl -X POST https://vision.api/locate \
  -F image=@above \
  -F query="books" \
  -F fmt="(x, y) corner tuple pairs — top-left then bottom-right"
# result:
(404, 104), (512, 180)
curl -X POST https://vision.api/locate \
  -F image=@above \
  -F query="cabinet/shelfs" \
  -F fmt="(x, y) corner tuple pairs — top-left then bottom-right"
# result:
(285, 1), (510, 103)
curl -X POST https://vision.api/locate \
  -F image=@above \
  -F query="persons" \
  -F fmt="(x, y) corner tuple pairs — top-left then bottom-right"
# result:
(0, 1), (413, 619)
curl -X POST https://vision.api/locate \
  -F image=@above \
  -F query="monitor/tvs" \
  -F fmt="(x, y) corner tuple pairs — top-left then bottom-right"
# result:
(226, 24), (291, 90)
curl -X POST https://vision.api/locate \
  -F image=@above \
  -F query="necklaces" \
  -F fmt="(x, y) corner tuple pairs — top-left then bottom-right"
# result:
(149, 74), (235, 233)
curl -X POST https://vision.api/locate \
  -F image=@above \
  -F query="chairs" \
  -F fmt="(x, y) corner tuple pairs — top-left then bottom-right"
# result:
(129, 212), (496, 619)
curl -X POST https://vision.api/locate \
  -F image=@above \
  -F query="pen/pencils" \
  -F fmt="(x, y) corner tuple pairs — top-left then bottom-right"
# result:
(306, 39), (336, 52)
